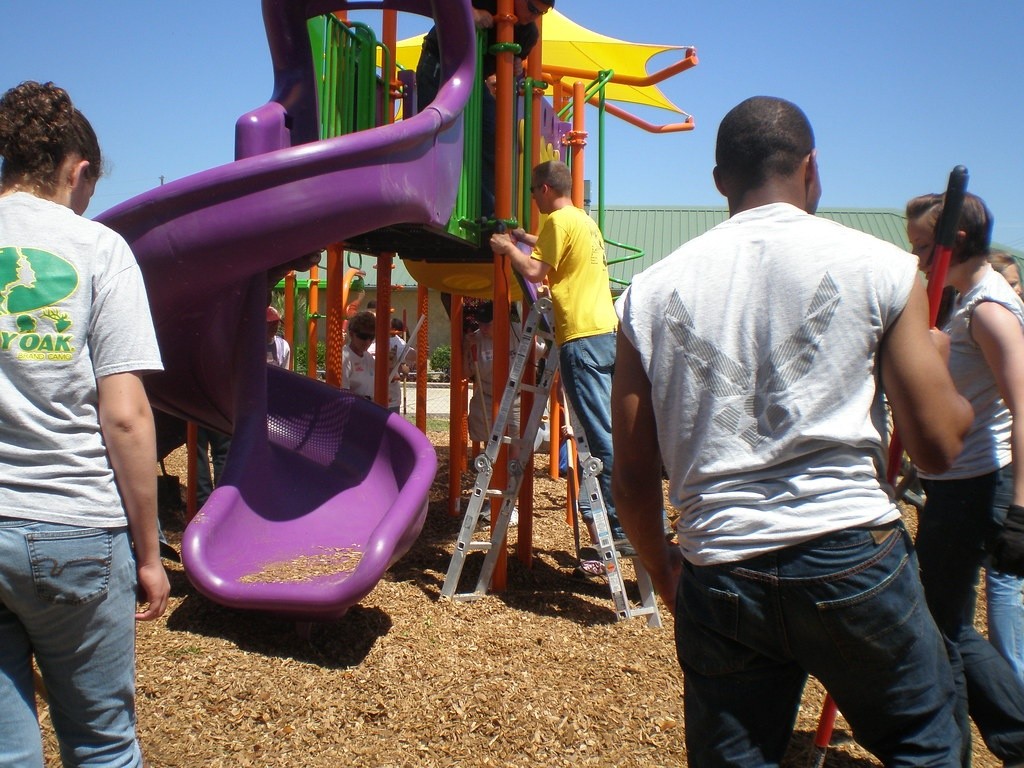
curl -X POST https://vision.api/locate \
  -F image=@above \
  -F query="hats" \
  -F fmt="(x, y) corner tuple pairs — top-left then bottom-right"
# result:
(474, 301), (494, 323)
(266, 305), (285, 325)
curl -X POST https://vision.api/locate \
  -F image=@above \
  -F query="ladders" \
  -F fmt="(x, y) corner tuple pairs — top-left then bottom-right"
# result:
(436, 298), (663, 630)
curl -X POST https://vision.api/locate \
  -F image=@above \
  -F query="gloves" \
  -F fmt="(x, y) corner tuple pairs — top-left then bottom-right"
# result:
(991, 505), (1023, 575)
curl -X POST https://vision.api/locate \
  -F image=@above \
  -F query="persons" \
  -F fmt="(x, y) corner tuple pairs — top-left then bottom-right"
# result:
(907, 192), (1024, 768)
(179, 421), (232, 523)
(464, 300), (547, 526)
(490, 161), (677, 557)
(267, 306), (291, 369)
(612, 97), (974, 768)
(0, 82), (171, 768)
(536, 372), (606, 577)
(341, 302), (416, 415)
(988, 249), (1024, 683)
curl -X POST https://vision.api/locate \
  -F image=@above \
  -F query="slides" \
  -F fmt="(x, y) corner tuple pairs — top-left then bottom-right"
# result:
(85, 0), (478, 627)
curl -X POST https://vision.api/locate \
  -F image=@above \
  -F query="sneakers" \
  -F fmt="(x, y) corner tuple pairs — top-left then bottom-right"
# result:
(664, 518), (675, 542)
(479, 503), (491, 522)
(507, 507), (518, 526)
(578, 537), (638, 560)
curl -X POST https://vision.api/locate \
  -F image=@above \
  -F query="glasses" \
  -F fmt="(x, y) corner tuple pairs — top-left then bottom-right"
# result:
(526, 0), (546, 16)
(354, 330), (376, 341)
(529, 183), (553, 195)
(474, 307), (489, 314)
